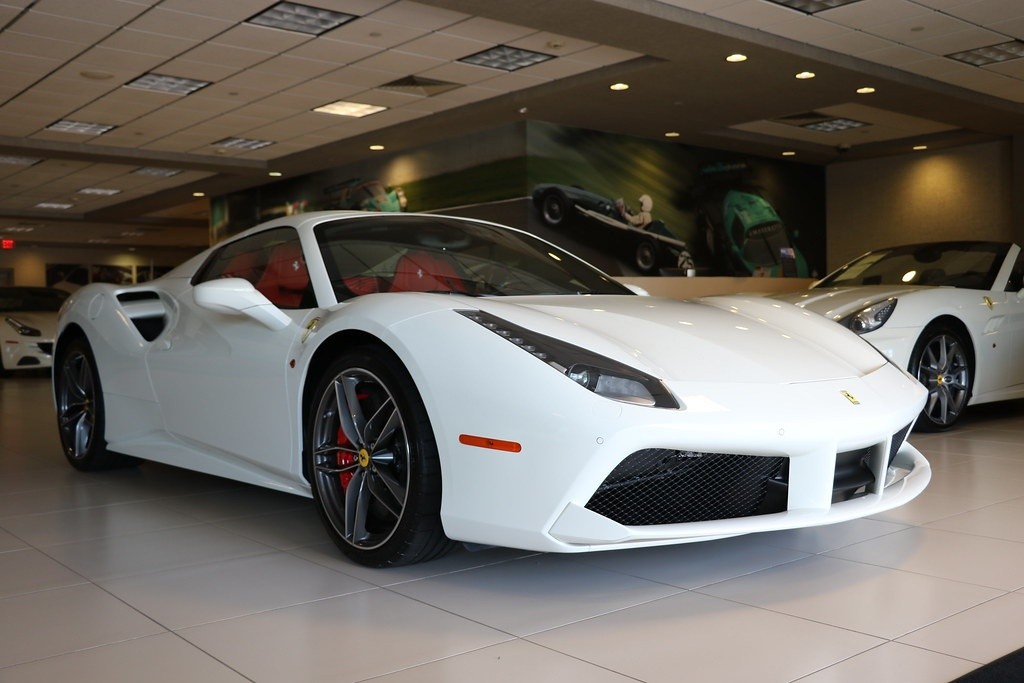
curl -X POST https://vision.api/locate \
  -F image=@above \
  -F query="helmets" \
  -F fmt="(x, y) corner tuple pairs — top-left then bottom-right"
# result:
(639, 194), (653, 212)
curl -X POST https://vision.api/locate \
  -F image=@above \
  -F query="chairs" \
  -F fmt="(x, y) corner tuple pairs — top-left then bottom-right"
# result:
(223, 244), (472, 307)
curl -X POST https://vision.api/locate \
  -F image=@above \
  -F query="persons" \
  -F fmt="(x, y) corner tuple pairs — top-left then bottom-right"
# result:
(617, 195), (654, 229)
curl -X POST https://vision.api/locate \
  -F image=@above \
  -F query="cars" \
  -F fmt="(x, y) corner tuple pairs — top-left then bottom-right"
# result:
(771, 239), (1024, 428)
(0, 282), (72, 373)
(533, 182), (695, 278)
(726, 189), (808, 283)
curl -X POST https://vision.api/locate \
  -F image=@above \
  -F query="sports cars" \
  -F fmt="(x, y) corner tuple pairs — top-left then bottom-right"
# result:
(44, 204), (933, 568)
